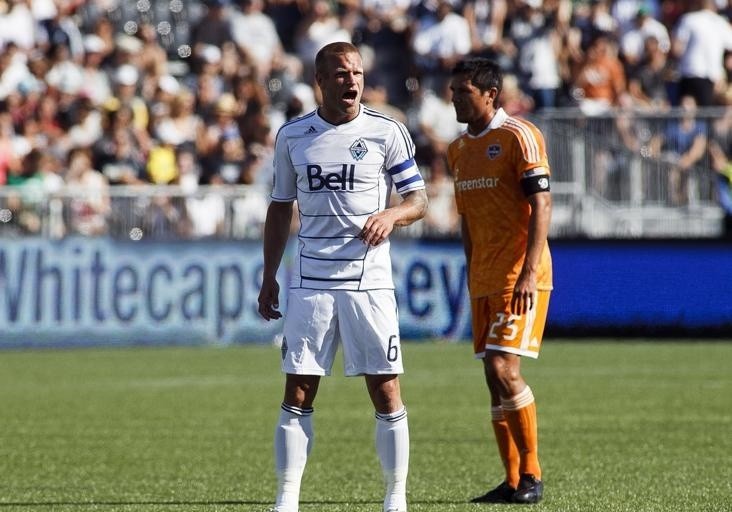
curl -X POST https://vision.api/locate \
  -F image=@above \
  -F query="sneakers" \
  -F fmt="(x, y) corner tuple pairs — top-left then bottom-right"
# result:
(515, 474), (543, 503)
(471, 482), (517, 503)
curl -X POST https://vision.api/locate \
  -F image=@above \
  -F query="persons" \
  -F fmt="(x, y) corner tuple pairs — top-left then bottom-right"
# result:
(0, 2), (732, 244)
(258, 40), (430, 512)
(442, 59), (556, 504)
